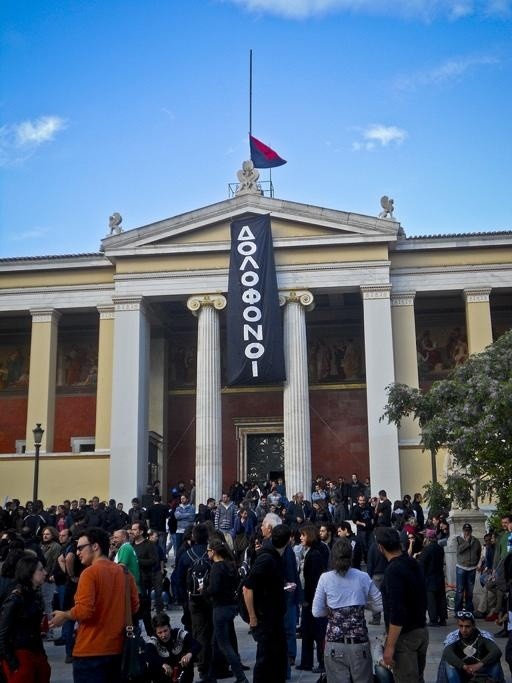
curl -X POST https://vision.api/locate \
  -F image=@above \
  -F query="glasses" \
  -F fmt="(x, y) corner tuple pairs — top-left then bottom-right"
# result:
(77, 542), (91, 552)
(458, 611), (475, 622)
(408, 537), (415, 540)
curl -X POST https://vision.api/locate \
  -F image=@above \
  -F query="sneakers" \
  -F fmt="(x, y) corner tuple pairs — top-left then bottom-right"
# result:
(440, 621), (449, 626)
(295, 664), (313, 671)
(368, 620), (381, 625)
(426, 622), (441, 627)
(311, 667), (327, 673)
(485, 612), (499, 622)
(473, 611), (484, 619)
(495, 630), (508, 638)
(232, 678), (249, 683)
(197, 678), (218, 683)
(231, 662), (251, 671)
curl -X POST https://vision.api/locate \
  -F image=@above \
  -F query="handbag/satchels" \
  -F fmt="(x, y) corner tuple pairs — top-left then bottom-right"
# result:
(122, 625), (149, 679)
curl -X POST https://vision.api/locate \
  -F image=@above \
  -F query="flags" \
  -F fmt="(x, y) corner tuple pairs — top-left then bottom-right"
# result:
(249, 133), (287, 168)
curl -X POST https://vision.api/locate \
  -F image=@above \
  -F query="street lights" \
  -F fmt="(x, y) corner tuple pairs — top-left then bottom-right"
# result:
(30, 420), (47, 504)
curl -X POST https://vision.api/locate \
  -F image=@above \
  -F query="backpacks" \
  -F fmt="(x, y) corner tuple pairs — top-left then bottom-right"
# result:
(238, 561), (251, 577)
(187, 559), (212, 597)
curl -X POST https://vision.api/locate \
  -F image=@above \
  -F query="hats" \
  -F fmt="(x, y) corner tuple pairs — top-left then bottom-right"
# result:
(463, 524), (472, 530)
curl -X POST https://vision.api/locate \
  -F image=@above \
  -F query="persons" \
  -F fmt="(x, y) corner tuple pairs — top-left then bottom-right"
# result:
(2, 474), (511, 683)
